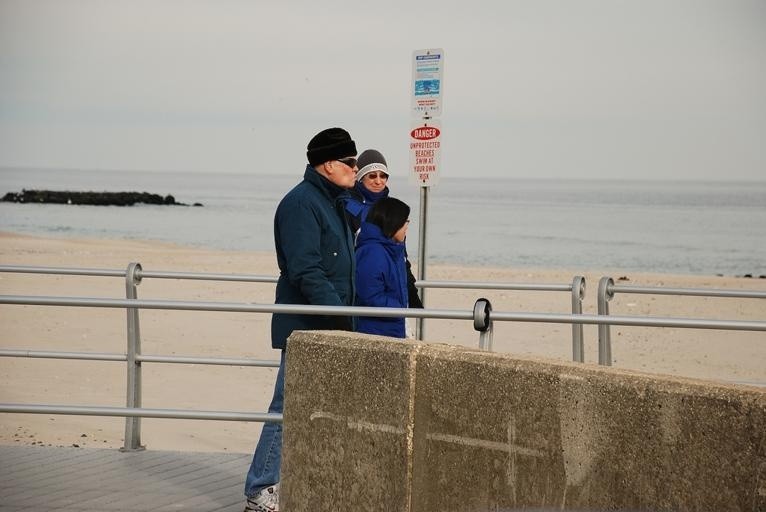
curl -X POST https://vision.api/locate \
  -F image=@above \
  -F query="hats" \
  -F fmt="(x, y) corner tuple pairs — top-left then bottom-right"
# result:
(356, 150), (389, 182)
(307, 128), (357, 165)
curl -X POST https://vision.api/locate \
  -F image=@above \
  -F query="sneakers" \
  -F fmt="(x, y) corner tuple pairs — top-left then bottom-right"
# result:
(245, 485), (279, 512)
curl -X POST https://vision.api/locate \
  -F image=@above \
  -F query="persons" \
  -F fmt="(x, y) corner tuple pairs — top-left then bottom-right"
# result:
(353, 196), (412, 338)
(242, 126), (360, 512)
(343, 150), (425, 308)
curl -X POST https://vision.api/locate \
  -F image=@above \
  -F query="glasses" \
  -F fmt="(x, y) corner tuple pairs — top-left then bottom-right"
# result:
(337, 158), (358, 168)
(368, 173), (386, 179)
